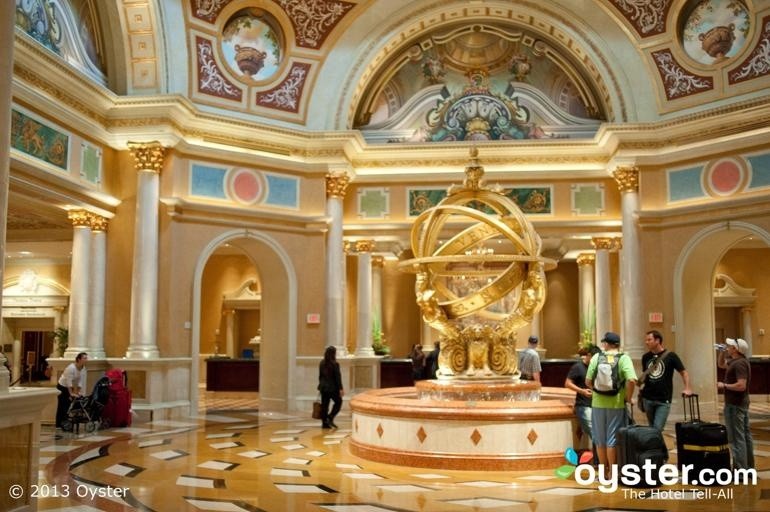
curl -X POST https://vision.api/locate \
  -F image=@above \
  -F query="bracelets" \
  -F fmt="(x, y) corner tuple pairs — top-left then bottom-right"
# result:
(724, 383), (727, 390)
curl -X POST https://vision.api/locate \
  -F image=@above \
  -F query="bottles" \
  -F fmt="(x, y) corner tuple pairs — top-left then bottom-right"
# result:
(0, 352), (10, 396)
(713, 343), (727, 353)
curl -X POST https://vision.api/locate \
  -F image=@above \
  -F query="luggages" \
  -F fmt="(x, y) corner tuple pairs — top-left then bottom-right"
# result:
(676, 394), (731, 487)
(103, 368), (131, 428)
(616, 400), (667, 489)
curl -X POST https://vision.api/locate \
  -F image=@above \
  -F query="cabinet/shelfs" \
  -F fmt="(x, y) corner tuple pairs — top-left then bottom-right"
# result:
(379, 356), (416, 389)
(715, 352), (770, 396)
(538, 359), (581, 389)
(203, 354), (260, 393)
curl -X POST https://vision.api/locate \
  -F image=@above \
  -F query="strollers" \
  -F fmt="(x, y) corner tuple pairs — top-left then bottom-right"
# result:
(60, 376), (111, 433)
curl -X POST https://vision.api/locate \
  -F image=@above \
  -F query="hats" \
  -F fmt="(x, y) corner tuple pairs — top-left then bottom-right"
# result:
(726, 338), (748, 355)
(602, 332), (620, 346)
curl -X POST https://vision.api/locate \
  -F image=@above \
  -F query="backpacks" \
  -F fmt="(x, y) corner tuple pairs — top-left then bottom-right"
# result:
(591, 350), (624, 396)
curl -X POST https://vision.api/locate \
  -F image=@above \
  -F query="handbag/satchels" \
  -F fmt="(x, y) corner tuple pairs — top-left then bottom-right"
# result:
(312, 401), (321, 419)
(107, 369), (123, 391)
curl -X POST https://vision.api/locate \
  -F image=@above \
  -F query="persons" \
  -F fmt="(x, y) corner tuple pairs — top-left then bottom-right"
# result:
(517, 335), (543, 384)
(585, 332), (639, 480)
(57, 352), (87, 428)
(408, 343), (425, 380)
(715, 338), (755, 482)
(564, 344), (609, 469)
(431, 341), (440, 378)
(0, 345), (12, 383)
(636, 330), (694, 434)
(317, 345), (345, 430)
